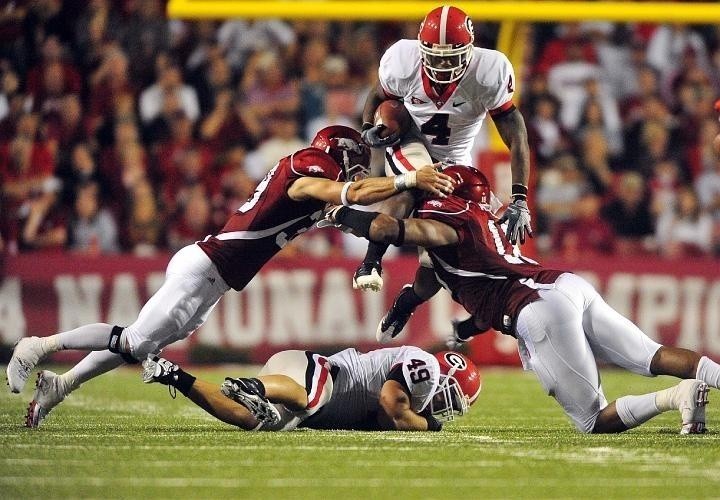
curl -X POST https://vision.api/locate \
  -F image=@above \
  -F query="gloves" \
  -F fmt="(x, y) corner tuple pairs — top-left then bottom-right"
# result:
(362, 122), (399, 147)
(445, 317), (470, 349)
(427, 416), (442, 431)
(497, 198), (533, 245)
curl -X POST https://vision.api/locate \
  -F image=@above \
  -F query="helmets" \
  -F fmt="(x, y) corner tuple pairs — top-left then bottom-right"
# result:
(429, 350), (481, 422)
(418, 6), (475, 84)
(421, 165), (491, 206)
(311, 125), (371, 181)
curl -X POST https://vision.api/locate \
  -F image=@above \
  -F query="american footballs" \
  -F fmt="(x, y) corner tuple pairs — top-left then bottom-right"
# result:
(374, 100), (410, 137)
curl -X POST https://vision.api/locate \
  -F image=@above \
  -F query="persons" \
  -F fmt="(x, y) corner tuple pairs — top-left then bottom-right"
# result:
(141, 344), (483, 432)
(316, 164), (720, 435)
(511, 20), (720, 258)
(6, 124), (456, 428)
(1, 0), (419, 261)
(351, 4), (536, 346)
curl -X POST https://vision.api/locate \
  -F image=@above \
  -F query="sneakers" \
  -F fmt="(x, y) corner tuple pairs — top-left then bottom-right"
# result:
(142, 353), (179, 388)
(6, 337), (42, 393)
(678, 379), (708, 434)
(377, 284), (419, 344)
(353, 260), (383, 289)
(27, 370), (62, 427)
(221, 377), (281, 427)
